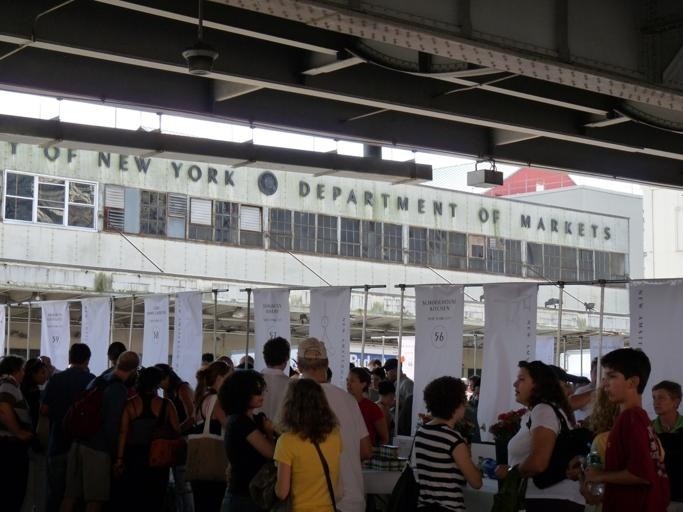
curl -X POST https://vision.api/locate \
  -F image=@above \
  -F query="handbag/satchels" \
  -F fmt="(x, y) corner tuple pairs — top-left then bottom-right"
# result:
(149, 396), (185, 467)
(528, 403), (596, 488)
(388, 429), (419, 512)
(182, 394), (229, 480)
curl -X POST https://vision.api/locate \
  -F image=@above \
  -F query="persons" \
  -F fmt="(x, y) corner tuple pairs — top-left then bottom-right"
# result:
(38, 343), (97, 511)
(216, 370), (282, 512)
(549, 364), (598, 413)
(494, 361), (588, 512)
(461, 376), (481, 412)
(60, 350), (139, 512)
(19, 357), (49, 512)
(579, 348), (671, 511)
(99, 341), (127, 377)
(371, 357), (414, 405)
(591, 378), (620, 468)
(0, 356), (34, 512)
(153, 364), (202, 512)
(410, 377), (484, 511)
(345, 367), (389, 447)
(253, 336), (292, 435)
(112, 367), (194, 512)
(190, 360), (234, 512)
(271, 377), (345, 512)
(295, 337), (369, 510)
(200, 352), (255, 370)
(650, 380), (683, 512)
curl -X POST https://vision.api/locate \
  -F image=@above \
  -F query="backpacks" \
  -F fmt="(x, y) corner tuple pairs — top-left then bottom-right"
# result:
(62, 373), (122, 440)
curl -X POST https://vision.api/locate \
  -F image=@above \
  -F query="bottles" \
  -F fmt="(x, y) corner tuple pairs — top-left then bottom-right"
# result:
(371, 444), (407, 472)
(585, 443), (605, 497)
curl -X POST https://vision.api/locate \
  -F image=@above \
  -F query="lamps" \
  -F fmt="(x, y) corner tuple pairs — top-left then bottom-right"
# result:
(182, 0), (219, 76)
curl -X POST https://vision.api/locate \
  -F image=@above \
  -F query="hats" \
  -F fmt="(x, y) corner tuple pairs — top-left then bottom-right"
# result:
(383, 358), (402, 370)
(297, 336), (328, 359)
(369, 367), (384, 375)
